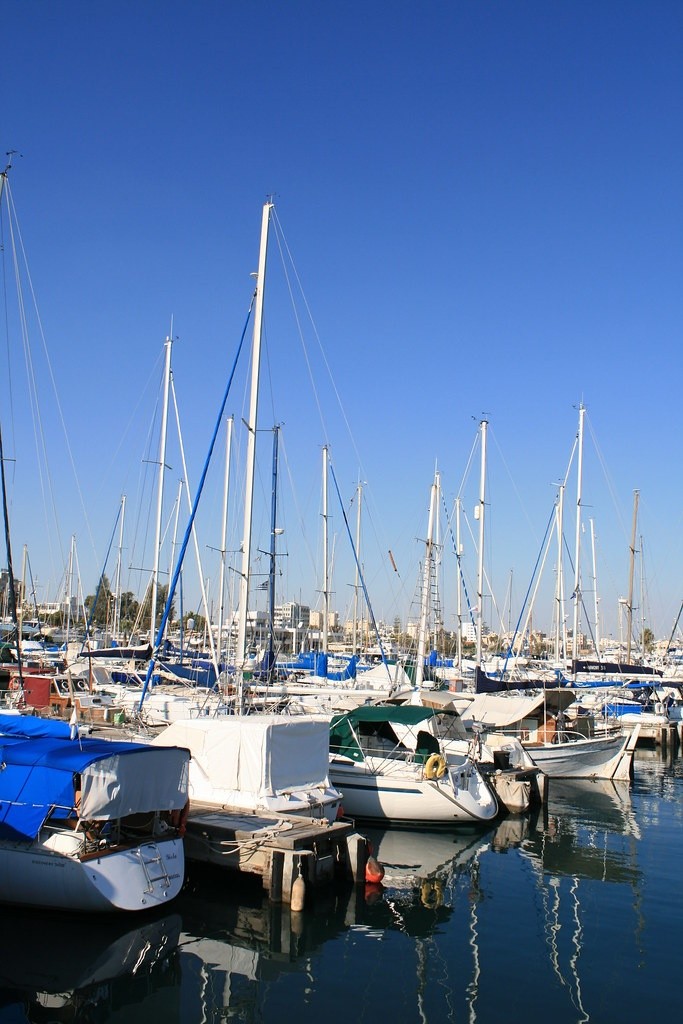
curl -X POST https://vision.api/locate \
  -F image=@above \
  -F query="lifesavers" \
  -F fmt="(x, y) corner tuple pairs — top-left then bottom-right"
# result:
(423, 752), (449, 780)
(654, 703), (665, 715)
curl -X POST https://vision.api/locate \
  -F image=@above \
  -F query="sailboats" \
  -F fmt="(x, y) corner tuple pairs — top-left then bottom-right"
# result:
(1, 146), (683, 915)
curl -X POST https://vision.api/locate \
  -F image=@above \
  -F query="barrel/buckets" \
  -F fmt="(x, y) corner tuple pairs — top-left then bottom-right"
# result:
(114, 713), (124, 724)
(493, 751), (510, 770)
